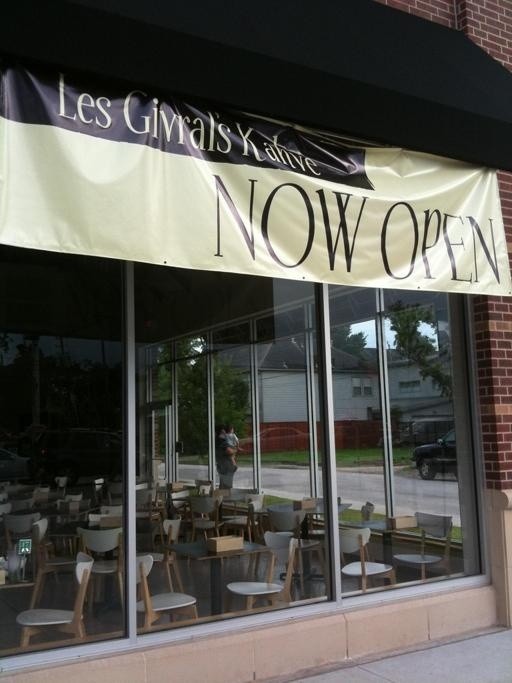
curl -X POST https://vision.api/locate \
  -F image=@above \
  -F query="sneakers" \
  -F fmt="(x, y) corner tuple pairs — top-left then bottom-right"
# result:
(233, 464), (237, 473)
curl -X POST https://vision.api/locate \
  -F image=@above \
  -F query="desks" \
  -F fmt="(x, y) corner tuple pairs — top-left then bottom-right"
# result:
(338, 515), (432, 585)
(168, 537), (270, 617)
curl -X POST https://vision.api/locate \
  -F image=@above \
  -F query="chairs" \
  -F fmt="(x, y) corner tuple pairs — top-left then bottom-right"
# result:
(338, 528), (393, 591)
(29, 517), (88, 610)
(390, 511), (453, 582)
(76, 526), (125, 610)
(125, 555), (201, 626)
(228, 530), (297, 611)
(144, 515), (185, 593)
(15, 550), (94, 648)
(2, 477), (374, 582)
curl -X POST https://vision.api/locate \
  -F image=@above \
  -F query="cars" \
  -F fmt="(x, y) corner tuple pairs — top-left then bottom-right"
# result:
(239, 426), (311, 451)
(0, 447), (31, 478)
(414, 427), (456, 479)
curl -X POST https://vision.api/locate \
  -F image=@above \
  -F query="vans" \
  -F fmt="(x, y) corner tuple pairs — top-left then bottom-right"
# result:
(21, 427), (122, 476)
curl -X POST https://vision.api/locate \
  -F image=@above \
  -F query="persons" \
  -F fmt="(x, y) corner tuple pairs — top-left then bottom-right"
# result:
(214, 422), (240, 490)
(225, 424), (240, 474)
